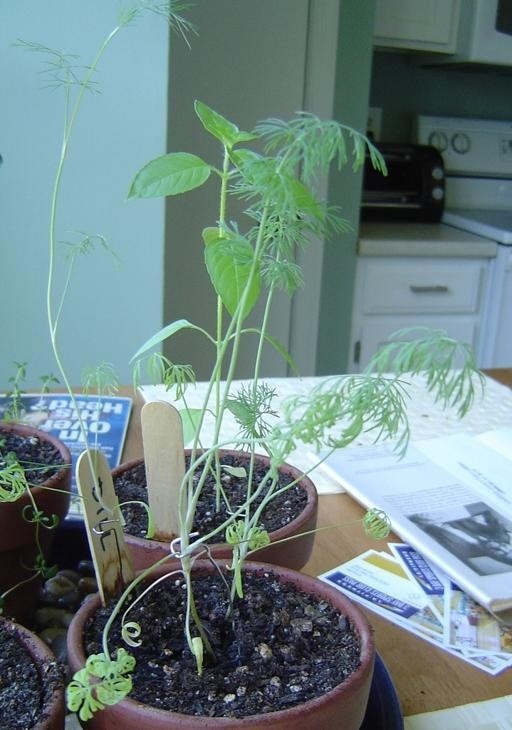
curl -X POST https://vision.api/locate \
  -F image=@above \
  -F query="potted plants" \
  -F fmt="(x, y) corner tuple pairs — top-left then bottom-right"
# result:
(67, 110), (488, 730)
(104, 99), (317, 573)
(1, 358), (74, 578)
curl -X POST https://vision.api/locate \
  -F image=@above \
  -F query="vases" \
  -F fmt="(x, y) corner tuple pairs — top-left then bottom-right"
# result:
(1, 612), (66, 729)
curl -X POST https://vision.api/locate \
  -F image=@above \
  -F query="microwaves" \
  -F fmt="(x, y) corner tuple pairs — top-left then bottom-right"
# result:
(361, 141), (445, 224)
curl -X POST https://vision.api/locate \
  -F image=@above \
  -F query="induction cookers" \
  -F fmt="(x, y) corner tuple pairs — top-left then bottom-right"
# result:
(446, 178), (512, 246)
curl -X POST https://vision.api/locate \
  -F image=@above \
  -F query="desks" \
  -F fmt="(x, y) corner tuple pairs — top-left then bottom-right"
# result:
(1, 367), (512, 728)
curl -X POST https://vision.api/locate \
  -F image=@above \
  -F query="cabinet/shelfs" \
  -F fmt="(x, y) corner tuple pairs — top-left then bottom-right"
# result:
(347, 254), (488, 376)
(372, 1), (459, 58)
